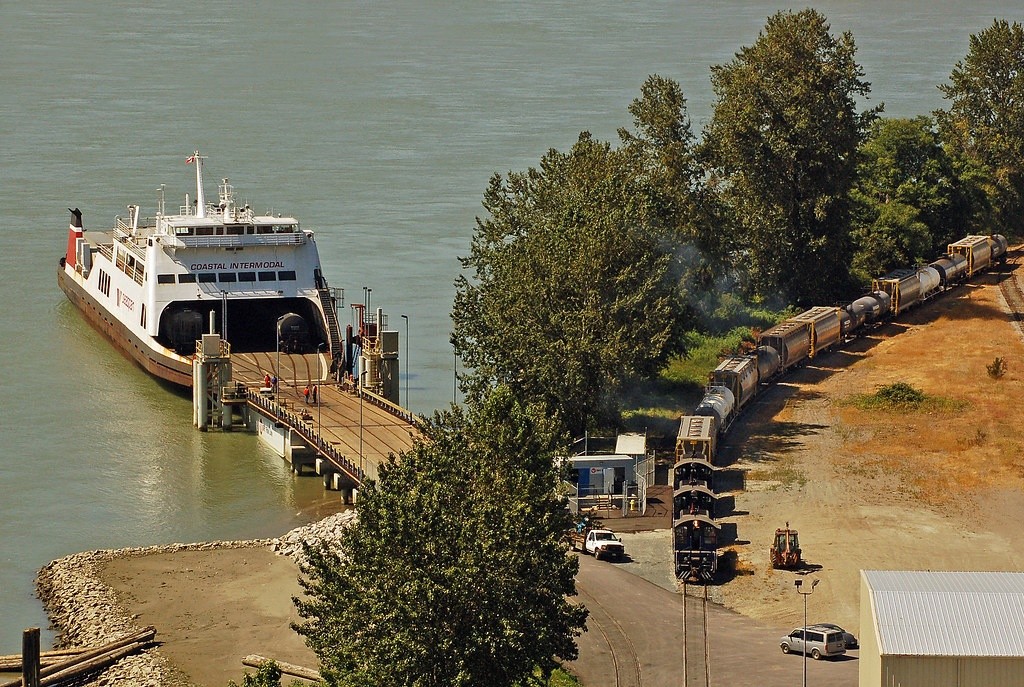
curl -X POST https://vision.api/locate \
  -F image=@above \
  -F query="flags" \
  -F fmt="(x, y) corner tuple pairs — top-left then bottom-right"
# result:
(185, 157), (194, 163)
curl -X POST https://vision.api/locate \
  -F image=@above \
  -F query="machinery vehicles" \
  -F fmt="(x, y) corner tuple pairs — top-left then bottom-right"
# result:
(770, 521), (802, 569)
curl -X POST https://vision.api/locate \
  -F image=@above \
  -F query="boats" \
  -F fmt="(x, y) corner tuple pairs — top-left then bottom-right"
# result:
(57, 149), (347, 388)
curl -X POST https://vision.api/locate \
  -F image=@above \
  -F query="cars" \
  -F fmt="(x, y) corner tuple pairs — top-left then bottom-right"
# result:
(807, 623), (857, 648)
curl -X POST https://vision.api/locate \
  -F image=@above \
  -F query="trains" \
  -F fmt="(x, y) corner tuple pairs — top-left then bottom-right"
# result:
(672, 232), (1007, 583)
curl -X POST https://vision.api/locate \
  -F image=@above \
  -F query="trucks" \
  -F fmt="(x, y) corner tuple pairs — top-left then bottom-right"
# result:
(570, 529), (625, 560)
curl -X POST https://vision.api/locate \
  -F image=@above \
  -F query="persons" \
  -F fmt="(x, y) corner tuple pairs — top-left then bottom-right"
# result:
(311, 384), (317, 403)
(304, 386), (310, 404)
(264, 374), (271, 388)
(271, 375), (277, 393)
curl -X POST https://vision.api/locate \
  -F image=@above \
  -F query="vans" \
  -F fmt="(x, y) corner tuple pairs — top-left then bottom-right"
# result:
(779, 626), (846, 660)
(259, 388), (273, 400)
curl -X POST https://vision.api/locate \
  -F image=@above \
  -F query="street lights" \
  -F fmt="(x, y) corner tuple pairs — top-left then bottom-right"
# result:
(360, 371), (367, 479)
(402, 316), (409, 410)
(794, 578), (820, 687)
(276, 317), (285, 424)
(317, 343), (324, 454)
